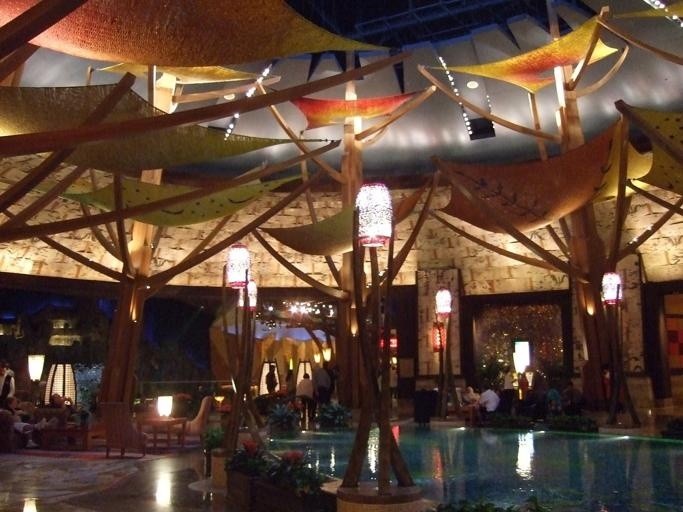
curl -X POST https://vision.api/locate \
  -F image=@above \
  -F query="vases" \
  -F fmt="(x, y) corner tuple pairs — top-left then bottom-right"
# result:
(254, 478), (338, 511)
(226, 468), (265, 512)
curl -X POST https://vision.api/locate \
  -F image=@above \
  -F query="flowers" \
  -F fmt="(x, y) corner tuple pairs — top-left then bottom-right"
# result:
(261, 449), (328, 496)
(224, 437), (281, 472)
(267, 401), (305, 432)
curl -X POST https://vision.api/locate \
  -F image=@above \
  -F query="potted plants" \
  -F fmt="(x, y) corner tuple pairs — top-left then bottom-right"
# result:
(200, 426), (225, 476)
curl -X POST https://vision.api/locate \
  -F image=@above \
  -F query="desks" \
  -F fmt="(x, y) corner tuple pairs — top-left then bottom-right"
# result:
(143, 417), (188, 448)
(39, 426), (93, 451)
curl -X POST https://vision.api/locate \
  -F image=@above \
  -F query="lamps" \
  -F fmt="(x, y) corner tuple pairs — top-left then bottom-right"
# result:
(237, 279), (258, 311)
(158, 394), (173, 418)
(227, 244), (251, 289)
(435, 286), (452, 319)
(432, 322), (446, 352)
(355, 181), (393, 248)
(26, 354), (45, 383)
(602, 272), (624, 304)
(43, 363), (78, 407)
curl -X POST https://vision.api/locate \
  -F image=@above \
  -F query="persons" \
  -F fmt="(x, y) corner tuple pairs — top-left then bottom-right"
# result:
(0, 363), (87, 450)
(461, 364), (580, 424)
(602, 364), (627, 415)
(265, 361), (336, 420)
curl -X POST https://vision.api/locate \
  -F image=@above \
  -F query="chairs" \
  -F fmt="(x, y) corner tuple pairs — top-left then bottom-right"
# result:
(99, 401), (148, 459)
(486, 389), (516, 415)
(0, 409), (29, 451)
(174, 396), (211, 448)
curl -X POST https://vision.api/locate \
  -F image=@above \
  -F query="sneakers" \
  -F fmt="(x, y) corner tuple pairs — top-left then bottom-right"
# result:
(38, 418), (47, 430)
(27, 441), (39, 448)
